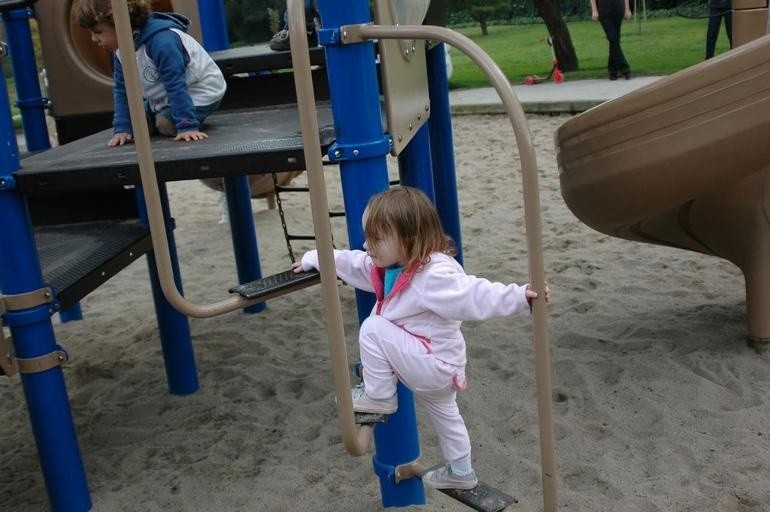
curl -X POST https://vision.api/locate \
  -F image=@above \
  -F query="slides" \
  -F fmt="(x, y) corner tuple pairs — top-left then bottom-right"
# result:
(35, 0), (308, 198)
(554, 0), (770, 341)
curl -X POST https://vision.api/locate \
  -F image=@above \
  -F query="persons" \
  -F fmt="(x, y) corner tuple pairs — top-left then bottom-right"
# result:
(264, 1), (319, 52)
(288, 182), (552, 492)
(67, 1), (229, 148)
(590, 0), (637, 81)
(698, 1), (734, 62)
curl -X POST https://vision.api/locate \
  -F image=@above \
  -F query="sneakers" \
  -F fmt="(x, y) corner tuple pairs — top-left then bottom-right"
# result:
(609, 70), (632, 80)
(335, 387), (399, 415)
(269, 28), (318, 52)
(422, 463), (478, 490)
